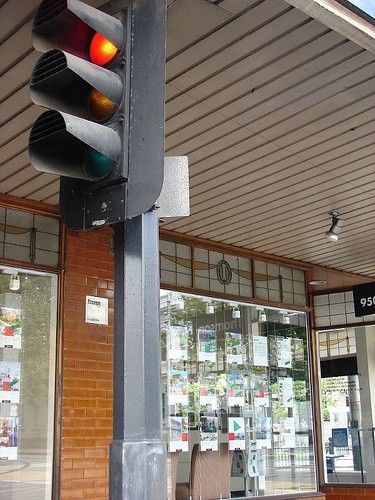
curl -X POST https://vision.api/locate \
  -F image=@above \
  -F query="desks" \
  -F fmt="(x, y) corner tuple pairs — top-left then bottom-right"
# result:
(326, 454), (344, 483)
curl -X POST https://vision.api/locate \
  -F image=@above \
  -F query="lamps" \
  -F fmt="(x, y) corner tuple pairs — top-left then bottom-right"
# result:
(282, 313), (290, 324)
(9, 275), (20, 291)
(176, 300), (184, 309)
(231, 307), (241, 320)
(324, 211), (344, 242)
(206, 303), (215, 314)
(259, 311), (267, 323)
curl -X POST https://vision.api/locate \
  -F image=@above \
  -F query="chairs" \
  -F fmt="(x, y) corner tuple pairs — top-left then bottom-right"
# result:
(176, 443), (234, 500)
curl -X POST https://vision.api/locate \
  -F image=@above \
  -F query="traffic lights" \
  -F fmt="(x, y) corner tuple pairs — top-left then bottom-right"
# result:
(27, 0), (130, 193)
